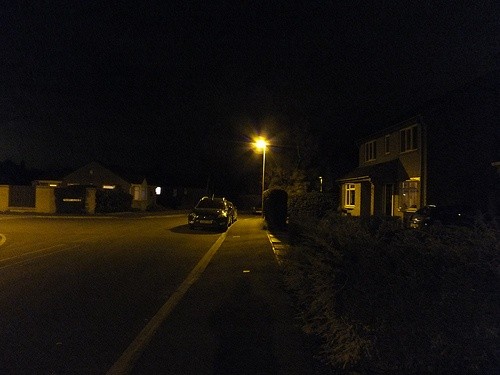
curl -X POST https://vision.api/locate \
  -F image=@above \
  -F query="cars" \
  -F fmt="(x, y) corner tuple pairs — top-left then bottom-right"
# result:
(227, 201), (237, 222)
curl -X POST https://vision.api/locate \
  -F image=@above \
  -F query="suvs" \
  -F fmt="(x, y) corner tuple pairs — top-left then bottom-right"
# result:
(408, 203), (479, 237)
(188, 196), (233, 231)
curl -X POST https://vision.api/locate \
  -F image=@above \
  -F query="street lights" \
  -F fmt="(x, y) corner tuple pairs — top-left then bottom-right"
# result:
(256, 140), (268, 217)
